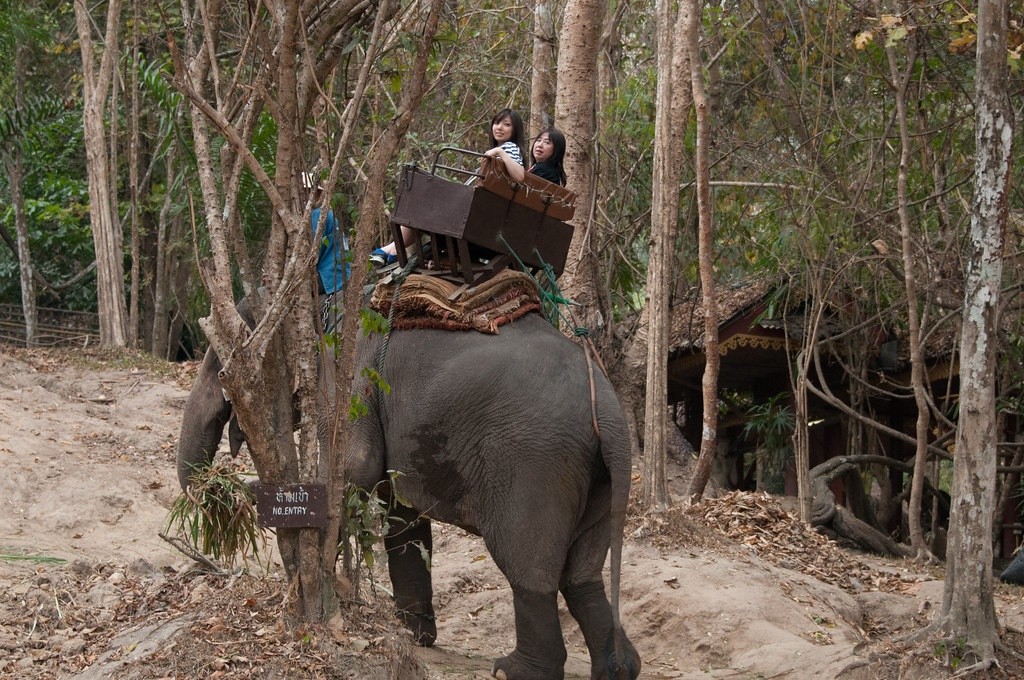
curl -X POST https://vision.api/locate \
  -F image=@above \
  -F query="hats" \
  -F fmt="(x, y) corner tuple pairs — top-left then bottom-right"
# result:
(302, 174), (323, 190)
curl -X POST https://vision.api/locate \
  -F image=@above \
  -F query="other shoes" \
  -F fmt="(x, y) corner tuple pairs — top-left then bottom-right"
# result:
(368, 249), (398, 267)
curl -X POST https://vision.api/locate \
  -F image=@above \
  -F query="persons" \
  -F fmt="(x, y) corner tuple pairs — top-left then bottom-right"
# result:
(526, 129), (569, 203)
(712, 437), (738, 491)
(369, 108), (529, 273)
(300, 166), (352, 298)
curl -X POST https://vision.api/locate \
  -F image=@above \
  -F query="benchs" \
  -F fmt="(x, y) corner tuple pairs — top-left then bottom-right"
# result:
(390, 146), (579, 303)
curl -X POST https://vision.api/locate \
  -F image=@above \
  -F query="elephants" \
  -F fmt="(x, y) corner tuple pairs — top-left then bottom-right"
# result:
(177, 271), (642, 680)
